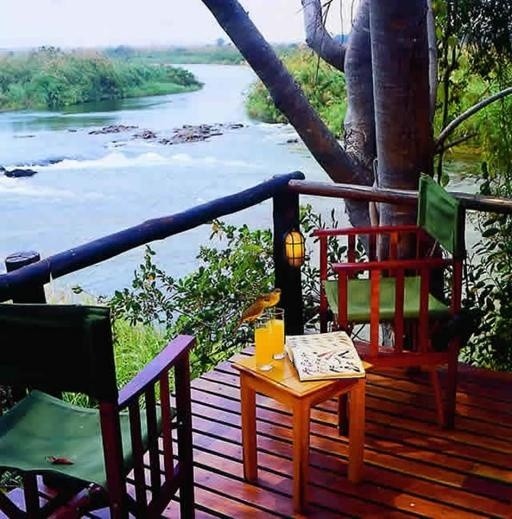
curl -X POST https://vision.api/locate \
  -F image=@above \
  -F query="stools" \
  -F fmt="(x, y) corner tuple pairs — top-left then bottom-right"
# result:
(230, 345), (373, 513)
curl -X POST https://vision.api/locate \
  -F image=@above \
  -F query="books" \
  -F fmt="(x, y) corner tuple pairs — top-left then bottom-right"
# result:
(284, 330), (367, 382)
(240, 345), (256, 356)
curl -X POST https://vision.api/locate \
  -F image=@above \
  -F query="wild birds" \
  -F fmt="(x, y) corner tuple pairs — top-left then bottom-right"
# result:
(233, 294), (269, 334)
(264, 288), (283, 308)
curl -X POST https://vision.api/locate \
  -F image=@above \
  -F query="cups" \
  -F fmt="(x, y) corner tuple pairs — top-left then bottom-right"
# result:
(254, 307), (286, 372)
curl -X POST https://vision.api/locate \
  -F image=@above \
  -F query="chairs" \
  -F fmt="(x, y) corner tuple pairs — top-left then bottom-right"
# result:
(314, 172), (465, 435)
(0, 304), (197, 519)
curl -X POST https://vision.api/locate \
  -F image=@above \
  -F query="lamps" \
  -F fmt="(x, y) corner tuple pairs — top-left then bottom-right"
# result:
(283, 227), (306, 270)
